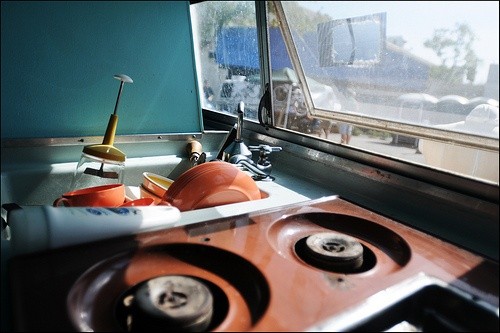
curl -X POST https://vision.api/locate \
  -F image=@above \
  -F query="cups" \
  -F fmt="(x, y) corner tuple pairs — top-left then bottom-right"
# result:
(52, 183), (125, 207)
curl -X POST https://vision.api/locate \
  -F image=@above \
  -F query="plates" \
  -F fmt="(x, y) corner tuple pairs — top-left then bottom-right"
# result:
(164, 162), (261, 212)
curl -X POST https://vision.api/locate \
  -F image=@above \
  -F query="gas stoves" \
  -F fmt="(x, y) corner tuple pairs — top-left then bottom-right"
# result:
(69, 197), (486, 332)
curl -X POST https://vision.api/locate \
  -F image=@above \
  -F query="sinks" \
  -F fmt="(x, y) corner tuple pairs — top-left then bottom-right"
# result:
(1, 150), (311, 247)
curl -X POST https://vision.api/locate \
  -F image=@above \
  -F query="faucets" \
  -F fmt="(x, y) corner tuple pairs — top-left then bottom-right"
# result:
(235, 101), (245, 142)
(228, 144), (283, 176)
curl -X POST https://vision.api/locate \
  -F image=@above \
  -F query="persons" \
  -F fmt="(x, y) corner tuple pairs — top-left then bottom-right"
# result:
(329, 79), (358, 144)
(203, 80), (216, 108)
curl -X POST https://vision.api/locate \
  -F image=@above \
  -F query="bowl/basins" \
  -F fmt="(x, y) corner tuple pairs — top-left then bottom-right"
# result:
(119, 172), (172, 207)
